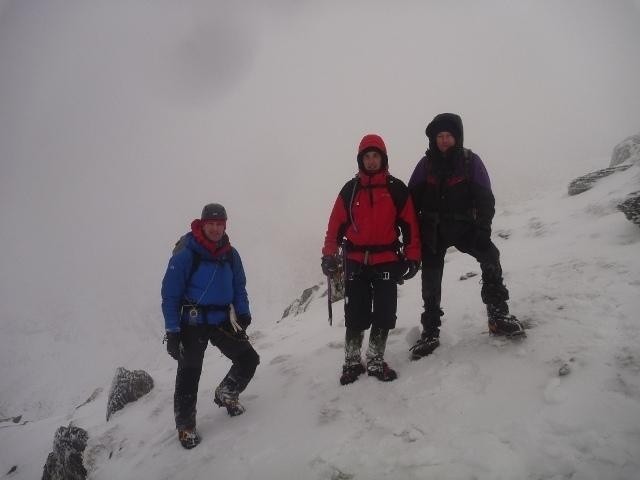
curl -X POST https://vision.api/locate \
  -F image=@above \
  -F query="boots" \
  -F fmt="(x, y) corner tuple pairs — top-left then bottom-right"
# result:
(366, 324), (399, 382)
(486, 303), (527, 338)
(338, 327), (365, 384)
(407, 323), (443, 359)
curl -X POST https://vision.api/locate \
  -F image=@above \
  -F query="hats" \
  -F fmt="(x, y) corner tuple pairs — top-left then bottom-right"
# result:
(200, 203), (227, 222)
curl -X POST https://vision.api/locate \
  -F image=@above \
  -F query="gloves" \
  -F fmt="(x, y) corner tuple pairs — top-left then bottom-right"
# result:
(475, 223), (491, 252)
(166, 330), (186, 359)
(320, 255), (342, 277)
(400, 259), (421, 279)
(236, 313), (252, 332)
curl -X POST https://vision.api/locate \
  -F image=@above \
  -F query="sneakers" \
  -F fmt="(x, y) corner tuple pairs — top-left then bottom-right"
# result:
(175, 420), (201, 449)
(214, 384), (244, 416)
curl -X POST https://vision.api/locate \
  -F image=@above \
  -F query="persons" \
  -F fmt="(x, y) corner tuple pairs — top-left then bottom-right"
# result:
(161, 203), (260, 450)
(408, 113), (524, 358)
(321, 134), (422, 386)
